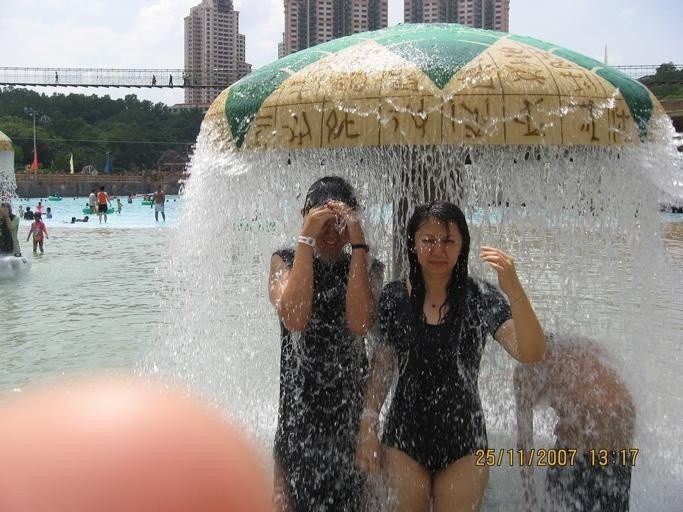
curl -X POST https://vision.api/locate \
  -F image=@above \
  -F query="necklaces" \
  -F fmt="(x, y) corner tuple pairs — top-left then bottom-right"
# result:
(431, 303), (436, 308)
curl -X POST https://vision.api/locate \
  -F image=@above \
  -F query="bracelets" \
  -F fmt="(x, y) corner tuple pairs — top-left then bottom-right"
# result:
(511, 292), (526, 306)
(351, 244), (370, 253)
(358, 409), (380, 421)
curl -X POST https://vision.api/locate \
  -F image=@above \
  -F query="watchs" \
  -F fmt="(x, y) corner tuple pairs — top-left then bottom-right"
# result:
(297, 235), (316, 247)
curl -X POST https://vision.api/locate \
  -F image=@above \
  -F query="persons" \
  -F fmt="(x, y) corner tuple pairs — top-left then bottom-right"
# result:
(169, 74), (173, 86)
(55, 71), (59, 84)
(0, 371), (274, 511)
(182, 72), (192, 85)
(382, 142), (469, 279)
(0, 186), (176, 257)
(513, 326), (635, 512)
(268, 175), (385, 512)
(152, 75), (156, 85)
(354, 200), (545, 512)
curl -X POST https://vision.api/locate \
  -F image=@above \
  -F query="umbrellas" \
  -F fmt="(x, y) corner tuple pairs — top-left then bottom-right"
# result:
(204, 21), (672, 151)
